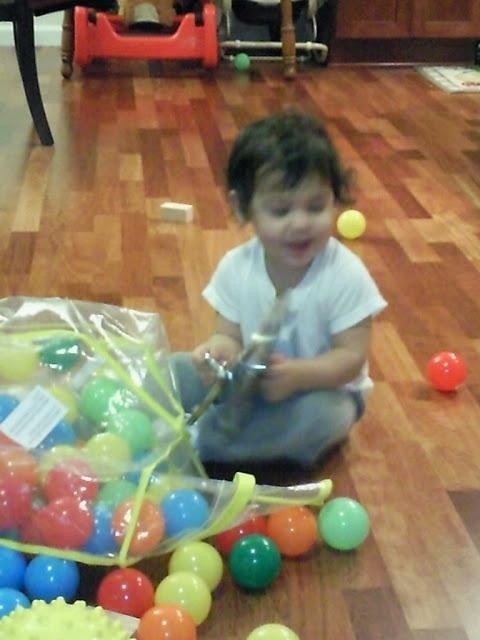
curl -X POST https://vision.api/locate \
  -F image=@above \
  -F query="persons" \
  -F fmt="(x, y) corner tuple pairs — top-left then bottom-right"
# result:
(141, 107), (389, 474)
